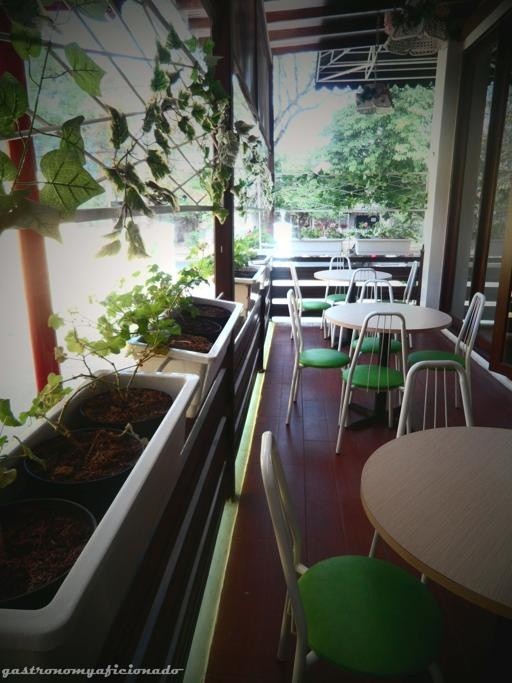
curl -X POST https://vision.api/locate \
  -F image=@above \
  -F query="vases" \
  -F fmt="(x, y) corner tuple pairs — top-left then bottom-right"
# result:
(300, 237), (412, 255)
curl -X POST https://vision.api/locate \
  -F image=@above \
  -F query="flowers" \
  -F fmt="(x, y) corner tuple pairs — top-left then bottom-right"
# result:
(299, 217), (419, 244)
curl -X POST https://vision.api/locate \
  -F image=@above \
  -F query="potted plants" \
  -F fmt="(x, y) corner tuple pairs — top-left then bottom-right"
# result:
(0, 226), (271, 683)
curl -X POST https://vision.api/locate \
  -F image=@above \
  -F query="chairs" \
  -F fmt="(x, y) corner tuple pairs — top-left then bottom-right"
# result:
(260, 255), (512, 683)
